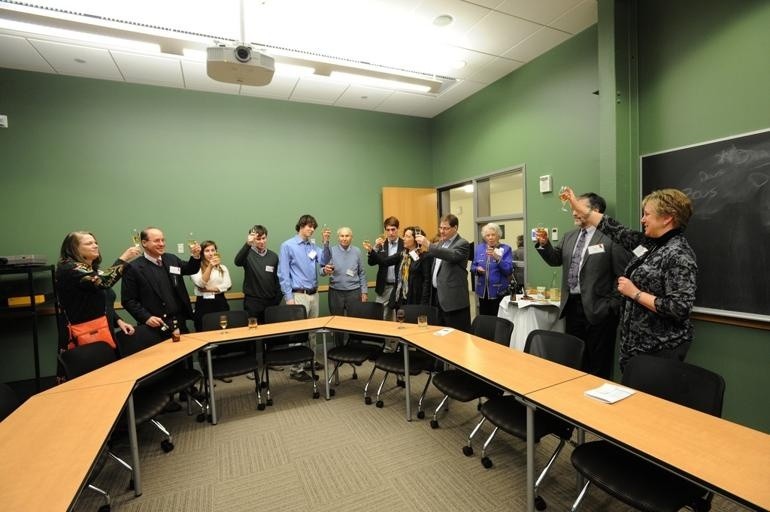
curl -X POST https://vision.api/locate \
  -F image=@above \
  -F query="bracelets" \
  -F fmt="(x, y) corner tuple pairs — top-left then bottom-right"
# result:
(582, 208), (593, 219)
(634, 290), (643, 301)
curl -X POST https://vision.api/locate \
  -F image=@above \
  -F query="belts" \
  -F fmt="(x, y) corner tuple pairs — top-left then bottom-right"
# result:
(293, 287), (318, 295)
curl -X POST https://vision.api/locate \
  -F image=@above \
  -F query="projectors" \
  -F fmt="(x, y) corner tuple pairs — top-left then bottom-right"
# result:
(207, 46), (275, 87)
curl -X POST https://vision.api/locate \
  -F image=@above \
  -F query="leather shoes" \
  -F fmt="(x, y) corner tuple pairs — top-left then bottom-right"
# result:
(179, 390), (209, 403)
(165, 403), (184, 413)
(303, 361), (324, 370)
(289, 369), (311, 381)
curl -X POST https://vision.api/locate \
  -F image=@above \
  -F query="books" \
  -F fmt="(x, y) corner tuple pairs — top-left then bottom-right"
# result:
(585, 382), (637, 406)
(6, 293), (54, 306)
(1, 254), (49, 267)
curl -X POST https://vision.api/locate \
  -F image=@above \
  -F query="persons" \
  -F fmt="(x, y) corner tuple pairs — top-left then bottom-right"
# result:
(415, 215), (471, 329)
(56, 231), (140, 384)
(374, 216), (404, 354)
(535, 192), (633, 335)
(565, 187), (699, 373)
(471, 223), (513, 315)
(234, 225), (284, 381)
(120, 227), (210, 405)
(277, 214), (333, 382)
(319, 227), (368, 348)
(362, 227), (434, 322)
(190, 240), (233, 386)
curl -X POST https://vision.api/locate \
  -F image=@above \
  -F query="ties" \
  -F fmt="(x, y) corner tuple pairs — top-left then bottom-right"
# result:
(157, 259), (162, 267)
(568, 230), (587, 289)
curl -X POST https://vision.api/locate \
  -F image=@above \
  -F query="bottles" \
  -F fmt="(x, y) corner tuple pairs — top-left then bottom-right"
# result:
(172, 315), (181, 342)
(510, 281), (517, 302)
(550, 271), (560, 301)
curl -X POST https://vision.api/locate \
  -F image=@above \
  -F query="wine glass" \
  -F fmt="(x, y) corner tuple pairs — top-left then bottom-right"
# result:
(329, 264), (336, 277)
(397, 310), (407, 328)
(537, 223), (545, 249)
(130, 229), (143, 256)
(187, 232), (198, 257)
(214, 252), (222, 271)
(364, 240), (372, 256)
(250, 225), (257, 248)
(320, 224), (330, 245)
(415, 228), (422, 250)
(220, 315), (228, 334)
(380, 234), (387, 252)
(486, 244), (494, 264)
(560, 185), (570, 212)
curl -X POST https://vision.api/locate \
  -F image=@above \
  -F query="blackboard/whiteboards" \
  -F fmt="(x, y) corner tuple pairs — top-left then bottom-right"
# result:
(640, 128), (770, 322)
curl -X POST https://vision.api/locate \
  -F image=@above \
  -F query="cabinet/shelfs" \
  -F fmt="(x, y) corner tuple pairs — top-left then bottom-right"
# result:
(1, 263), (60, 393)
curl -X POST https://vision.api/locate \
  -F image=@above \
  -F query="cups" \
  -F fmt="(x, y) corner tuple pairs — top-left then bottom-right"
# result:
(537, 287), (546, 301)
(417, 315), (428, 327)
(248, 318), (258, 331)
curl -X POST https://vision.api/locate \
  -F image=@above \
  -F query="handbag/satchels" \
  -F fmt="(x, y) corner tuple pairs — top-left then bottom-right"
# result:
(65, 315), (116, 352)
(495, 284), (523, 306)
